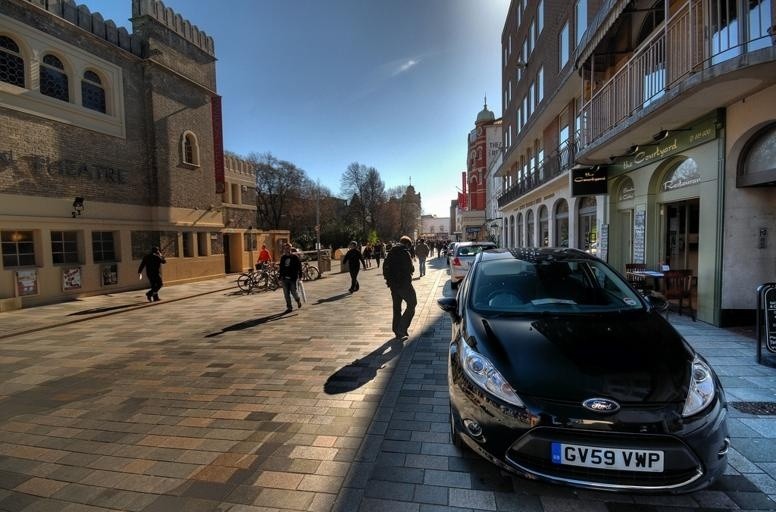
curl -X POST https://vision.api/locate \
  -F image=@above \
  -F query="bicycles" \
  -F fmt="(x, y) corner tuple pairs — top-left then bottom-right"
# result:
(237, 259), (320, 292)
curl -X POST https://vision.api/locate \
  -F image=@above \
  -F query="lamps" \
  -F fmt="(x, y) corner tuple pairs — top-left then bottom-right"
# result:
(591, 127), (700, 175)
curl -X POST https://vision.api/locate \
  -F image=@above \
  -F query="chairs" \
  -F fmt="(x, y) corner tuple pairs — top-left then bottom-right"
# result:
(625, 262), (695, 323)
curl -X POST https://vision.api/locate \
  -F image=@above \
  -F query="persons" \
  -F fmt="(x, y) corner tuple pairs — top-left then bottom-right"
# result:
(363, 237), (451, 278)
(278, 242), (303, 312)
(138, 245), (166, 303)
(383, 235), (417, 340)
(257, 243), (272, 273)
(290, 247), (307, 304)
(342, 240), (367, 294)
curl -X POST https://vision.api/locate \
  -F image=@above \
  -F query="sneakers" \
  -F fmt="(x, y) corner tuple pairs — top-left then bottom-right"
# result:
(393, 327), (411, 342)
(284, 301), (302, 313)
(153, 297), (161, 302)
(348, 287), (360, 294)
(145, 292), (152, 302)
